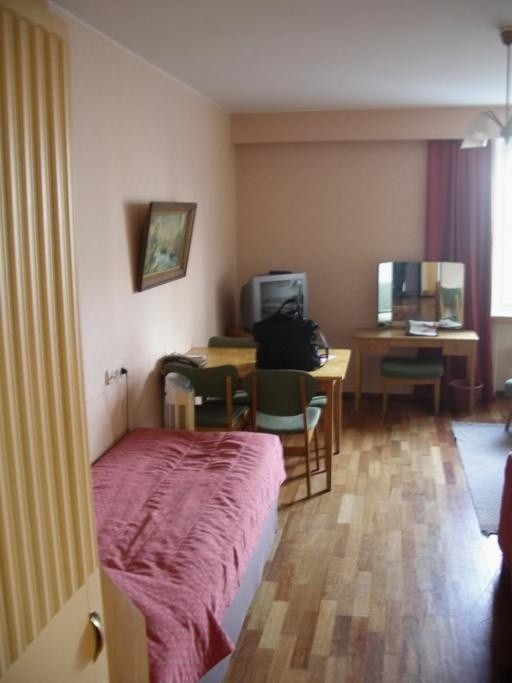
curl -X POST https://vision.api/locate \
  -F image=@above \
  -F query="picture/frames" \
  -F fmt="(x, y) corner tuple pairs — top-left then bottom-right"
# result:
(138, 202), (196, 289)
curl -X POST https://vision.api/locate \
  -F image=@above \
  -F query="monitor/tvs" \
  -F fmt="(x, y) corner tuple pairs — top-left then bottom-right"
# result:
(240, 272), (309, 332)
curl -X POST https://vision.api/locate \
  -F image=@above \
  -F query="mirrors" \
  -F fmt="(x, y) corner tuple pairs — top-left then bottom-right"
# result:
(377, 261), (465, 328)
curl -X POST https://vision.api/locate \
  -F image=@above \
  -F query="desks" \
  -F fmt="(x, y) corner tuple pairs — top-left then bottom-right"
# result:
(354, 327), (480, 412)
(183, 347), (352, 492)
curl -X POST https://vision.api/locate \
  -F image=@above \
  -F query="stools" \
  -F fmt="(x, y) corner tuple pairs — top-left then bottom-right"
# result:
(504, 377), (512, 431)
(381, 357), (443, 414)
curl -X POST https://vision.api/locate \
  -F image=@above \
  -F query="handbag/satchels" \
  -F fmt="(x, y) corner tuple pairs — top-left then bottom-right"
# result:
(250, 298), (329, 372)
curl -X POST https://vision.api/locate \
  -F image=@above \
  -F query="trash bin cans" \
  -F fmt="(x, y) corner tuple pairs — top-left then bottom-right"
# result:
(449, 379), (485, 411)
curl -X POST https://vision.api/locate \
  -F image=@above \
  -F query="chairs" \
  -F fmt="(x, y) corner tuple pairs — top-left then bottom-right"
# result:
(309, 390), (327, 407)
(162, 363), (252, 430)
(202, 335), (255, 403)
(240, 368), (321, 499)
(440, 286), (463, 322)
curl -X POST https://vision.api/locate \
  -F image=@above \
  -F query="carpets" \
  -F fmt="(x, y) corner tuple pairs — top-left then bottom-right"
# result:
(451, 417), (512, 537)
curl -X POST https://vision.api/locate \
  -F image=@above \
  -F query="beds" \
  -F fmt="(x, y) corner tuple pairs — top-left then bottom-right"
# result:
(88, 428), (286, 683)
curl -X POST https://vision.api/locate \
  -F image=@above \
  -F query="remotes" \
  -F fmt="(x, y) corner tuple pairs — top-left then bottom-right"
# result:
(268, 270), (291, 275)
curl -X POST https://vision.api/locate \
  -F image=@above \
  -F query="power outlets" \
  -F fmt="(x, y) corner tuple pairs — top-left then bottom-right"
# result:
(106, 366), (128, 384)
